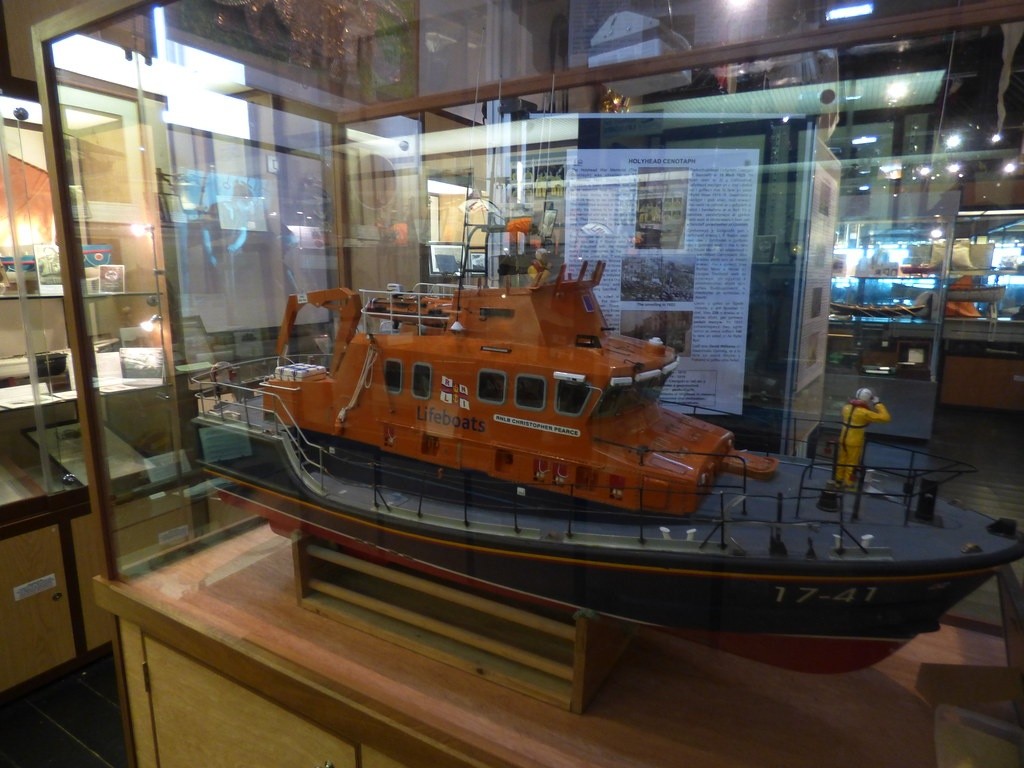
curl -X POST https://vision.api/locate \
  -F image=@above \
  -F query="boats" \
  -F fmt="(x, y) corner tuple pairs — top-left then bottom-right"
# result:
(186, 213), (1024, 677)
(828, 299), (925, 318)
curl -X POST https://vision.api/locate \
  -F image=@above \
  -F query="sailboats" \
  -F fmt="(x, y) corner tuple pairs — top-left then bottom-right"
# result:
(891, 241), (1007, 302)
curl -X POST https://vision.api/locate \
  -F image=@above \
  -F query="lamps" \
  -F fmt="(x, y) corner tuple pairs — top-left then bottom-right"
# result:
(128, 222), (172, 384)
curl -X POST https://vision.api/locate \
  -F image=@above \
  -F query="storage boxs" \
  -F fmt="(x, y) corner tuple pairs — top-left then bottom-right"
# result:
(81, 244), (113, 268)
(0, 255), (37, 272)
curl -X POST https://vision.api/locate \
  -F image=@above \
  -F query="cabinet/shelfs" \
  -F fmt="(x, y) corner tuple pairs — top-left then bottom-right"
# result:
(0, 66), (210, 705)
(819, 190), (962, 437)
(936, 210), (1024, 413)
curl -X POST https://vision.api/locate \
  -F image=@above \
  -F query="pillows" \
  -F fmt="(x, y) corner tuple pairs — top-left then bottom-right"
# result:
(927, 240), (995, 271)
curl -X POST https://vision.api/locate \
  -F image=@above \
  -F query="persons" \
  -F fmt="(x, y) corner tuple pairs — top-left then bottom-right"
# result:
(835, 387), (892, 491)
(529, 247), (552, 288)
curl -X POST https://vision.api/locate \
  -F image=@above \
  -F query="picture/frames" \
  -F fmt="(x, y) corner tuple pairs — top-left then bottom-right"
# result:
(32, 243), (64, 297)
